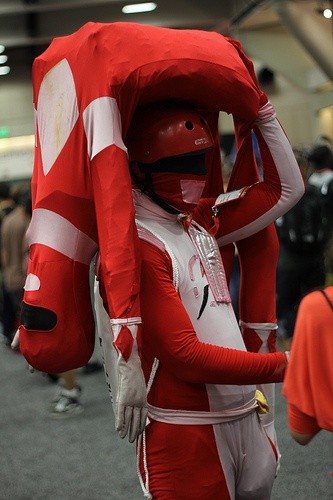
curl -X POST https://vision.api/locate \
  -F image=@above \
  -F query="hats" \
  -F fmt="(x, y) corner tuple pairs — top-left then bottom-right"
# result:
(306, 146), (333, 169)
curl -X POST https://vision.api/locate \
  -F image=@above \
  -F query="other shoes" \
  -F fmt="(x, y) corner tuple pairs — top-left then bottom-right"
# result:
(48, 389), (84, 420)
(51, 384), (84, 402)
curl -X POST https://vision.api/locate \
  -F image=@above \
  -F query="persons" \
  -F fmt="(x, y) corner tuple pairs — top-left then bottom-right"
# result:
(0, 40), (333, 500)
(11, 21), (282, 479)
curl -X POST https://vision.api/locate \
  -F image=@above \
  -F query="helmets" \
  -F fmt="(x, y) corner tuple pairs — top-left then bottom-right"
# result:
(127, 107), (216, 164)
